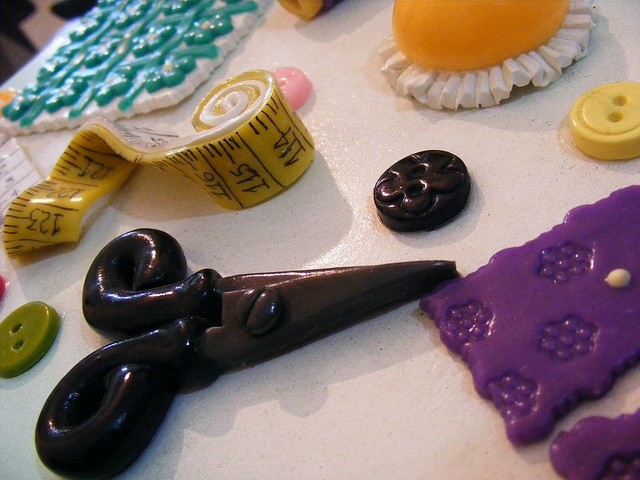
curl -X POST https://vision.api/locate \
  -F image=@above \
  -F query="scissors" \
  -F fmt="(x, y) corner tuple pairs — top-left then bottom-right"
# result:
(34, 227), (456, 476)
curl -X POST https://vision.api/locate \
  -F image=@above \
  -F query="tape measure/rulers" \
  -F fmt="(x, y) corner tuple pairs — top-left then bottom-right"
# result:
(3, 70), (315, 259)
